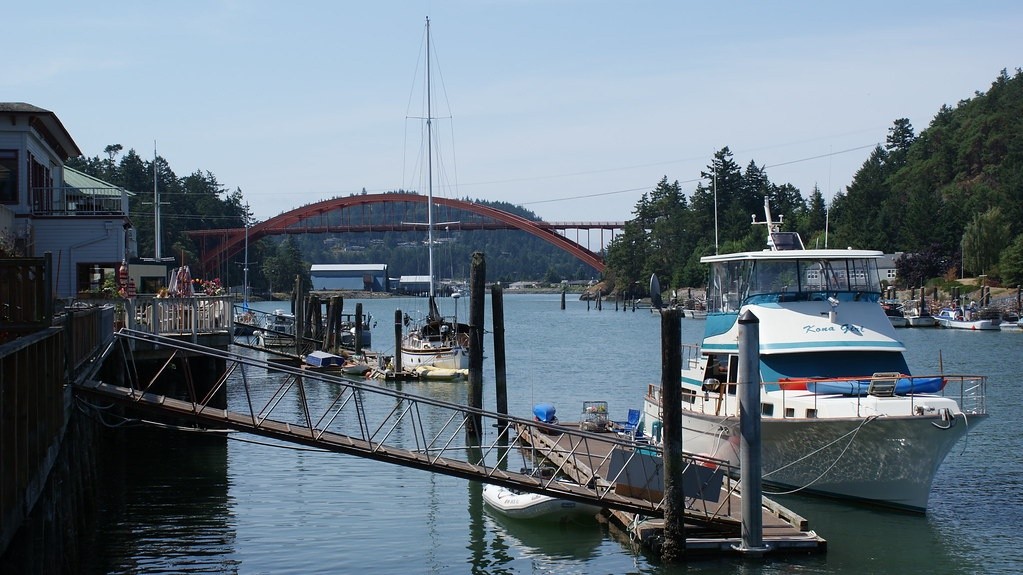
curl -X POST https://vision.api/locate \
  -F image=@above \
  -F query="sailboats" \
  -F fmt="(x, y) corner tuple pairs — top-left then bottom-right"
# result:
(393, 15), (472, 372)
(231, 200), (257, 336)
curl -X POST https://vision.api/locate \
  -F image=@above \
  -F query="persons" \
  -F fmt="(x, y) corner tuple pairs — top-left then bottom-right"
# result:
(970, 298), (976, 309)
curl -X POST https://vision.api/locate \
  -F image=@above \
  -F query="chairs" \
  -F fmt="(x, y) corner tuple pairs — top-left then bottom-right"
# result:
(612, 409), (640, 446)
(632, 421), (663, 456)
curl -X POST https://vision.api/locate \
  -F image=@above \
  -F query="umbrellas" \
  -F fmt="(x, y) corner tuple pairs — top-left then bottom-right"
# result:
(167, 265), (196, 298)
(119, 259), (128, 288)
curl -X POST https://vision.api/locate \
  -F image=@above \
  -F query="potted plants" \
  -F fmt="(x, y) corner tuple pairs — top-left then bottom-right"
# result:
(113, 304), (124, 331)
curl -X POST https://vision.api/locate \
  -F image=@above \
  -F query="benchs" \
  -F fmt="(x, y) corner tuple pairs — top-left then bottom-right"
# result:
(582, 401), (610, 422)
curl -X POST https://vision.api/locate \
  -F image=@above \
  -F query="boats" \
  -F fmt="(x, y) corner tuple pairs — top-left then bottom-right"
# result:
(902, 300), (936, 327)
(340, 356), (367, 375)
(931, 295), (1002, 331)
(403, 363), (469, 380)
(322, 313), (373, 349)
(877, 300), (906, 328)
(251, 309), (296, 348)
(649, 292), (739, 318)
(642, 145), (991, 513)
(480, 467), (605, 530)
